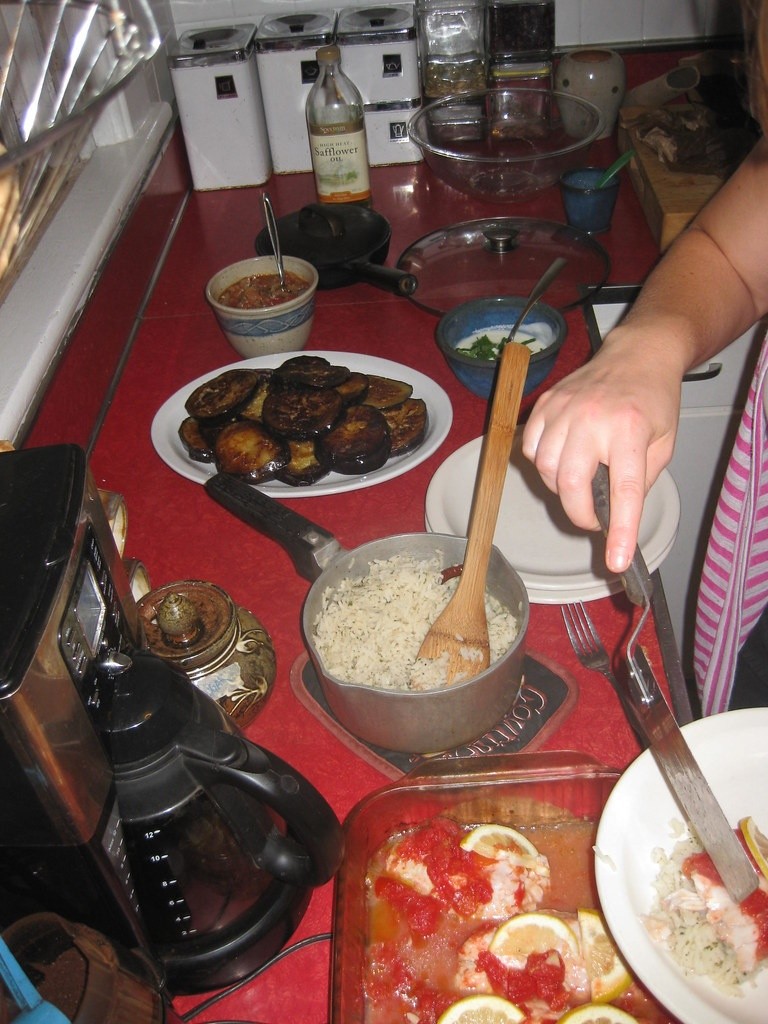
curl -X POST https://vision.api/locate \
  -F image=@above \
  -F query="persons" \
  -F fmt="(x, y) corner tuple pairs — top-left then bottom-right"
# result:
(520, 1), (767, 726)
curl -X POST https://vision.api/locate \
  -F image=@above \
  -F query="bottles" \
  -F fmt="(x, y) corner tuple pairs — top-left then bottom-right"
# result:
(130, 580), (276, 736)
(304, 45), (372, 210)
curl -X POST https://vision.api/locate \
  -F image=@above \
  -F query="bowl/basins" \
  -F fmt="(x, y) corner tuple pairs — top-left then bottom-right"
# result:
(416, 0), (624, 142)
(326, 751), (683, 1024)
(204, 255), (319, 360)
(436, 296), (568, 401)
(408, 86), (606, 206)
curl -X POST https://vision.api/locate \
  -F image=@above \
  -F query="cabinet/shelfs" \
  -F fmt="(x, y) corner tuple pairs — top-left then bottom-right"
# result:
(593, 303), (763, 661)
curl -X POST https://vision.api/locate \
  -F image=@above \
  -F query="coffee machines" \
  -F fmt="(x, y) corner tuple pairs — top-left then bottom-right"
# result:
(0, 442), (345, 997)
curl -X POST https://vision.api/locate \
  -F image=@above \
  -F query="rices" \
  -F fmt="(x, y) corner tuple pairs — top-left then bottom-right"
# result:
(645, 818), (767, 997)
(313, 550), (522, 695)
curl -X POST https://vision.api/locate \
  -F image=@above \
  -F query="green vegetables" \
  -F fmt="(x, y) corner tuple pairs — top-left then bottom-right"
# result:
(456, 334), (544, 362)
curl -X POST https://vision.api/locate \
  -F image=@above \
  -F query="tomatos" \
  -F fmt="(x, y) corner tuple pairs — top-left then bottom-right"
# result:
(361, 817), (571, 1023)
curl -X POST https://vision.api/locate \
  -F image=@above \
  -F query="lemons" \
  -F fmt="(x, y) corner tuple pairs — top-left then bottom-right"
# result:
(739, 815), (767, 884)
(433, 819), (643, 1024)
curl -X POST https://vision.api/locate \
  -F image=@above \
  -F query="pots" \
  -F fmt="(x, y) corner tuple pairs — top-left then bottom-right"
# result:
(202, 473), (530, 753)
(255, 203), (419, 306)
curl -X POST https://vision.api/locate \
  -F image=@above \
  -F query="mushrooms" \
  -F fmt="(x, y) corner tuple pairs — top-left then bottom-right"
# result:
(177, 356), (429, 485)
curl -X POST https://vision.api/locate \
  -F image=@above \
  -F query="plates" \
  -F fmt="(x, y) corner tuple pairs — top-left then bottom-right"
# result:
(423, 422), (682, 605)
(591, 707), (768, 1024)
(150, 349), (455, 499)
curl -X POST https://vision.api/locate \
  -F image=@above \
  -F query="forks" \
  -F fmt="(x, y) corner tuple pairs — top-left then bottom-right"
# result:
(559, 599), (654, 754)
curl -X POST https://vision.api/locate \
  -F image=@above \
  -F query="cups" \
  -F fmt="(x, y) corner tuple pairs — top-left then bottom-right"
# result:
(559, 168), (620, 237)
(0, 912), (164, 1024)
